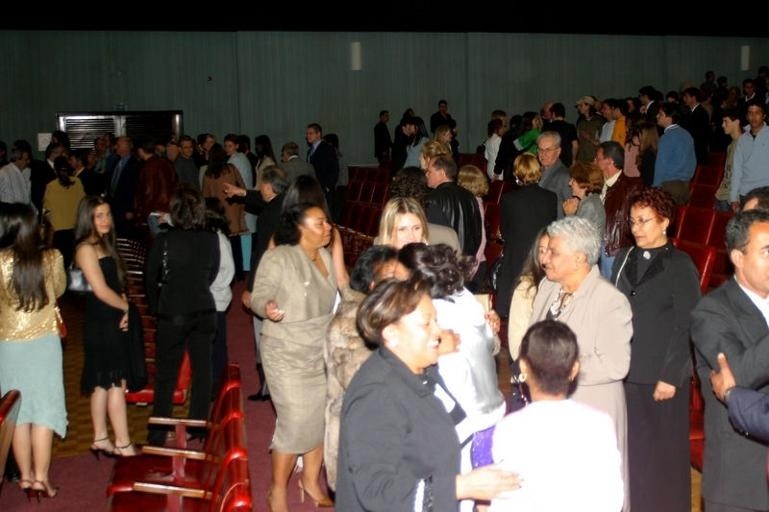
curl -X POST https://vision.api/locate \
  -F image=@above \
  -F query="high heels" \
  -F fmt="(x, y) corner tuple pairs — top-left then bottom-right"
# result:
(19, 479), (36, 502)
(32, 479), (60, 503)
(296, 477), (334, 507)
(90, 437), (134, 460)
(264, 484), (292, 511)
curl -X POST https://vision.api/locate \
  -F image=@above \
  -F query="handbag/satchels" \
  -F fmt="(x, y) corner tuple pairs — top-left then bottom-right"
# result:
(65, 268), (95, 292)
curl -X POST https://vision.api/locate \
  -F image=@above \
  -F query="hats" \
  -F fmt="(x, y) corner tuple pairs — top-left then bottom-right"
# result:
(575, 96), (595, 107)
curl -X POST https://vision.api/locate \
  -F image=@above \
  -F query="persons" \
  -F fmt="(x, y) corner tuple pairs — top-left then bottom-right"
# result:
(0, 64), (768, 512)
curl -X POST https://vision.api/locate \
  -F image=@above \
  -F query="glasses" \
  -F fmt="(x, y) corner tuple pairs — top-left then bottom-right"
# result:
(628, 214), (660, 226)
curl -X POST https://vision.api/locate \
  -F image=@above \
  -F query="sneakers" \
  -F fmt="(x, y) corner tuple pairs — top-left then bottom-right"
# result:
(247, 392), (273, 402)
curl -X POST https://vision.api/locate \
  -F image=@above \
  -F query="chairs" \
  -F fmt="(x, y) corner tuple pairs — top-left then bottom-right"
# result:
(1, 386), (22, 494)
